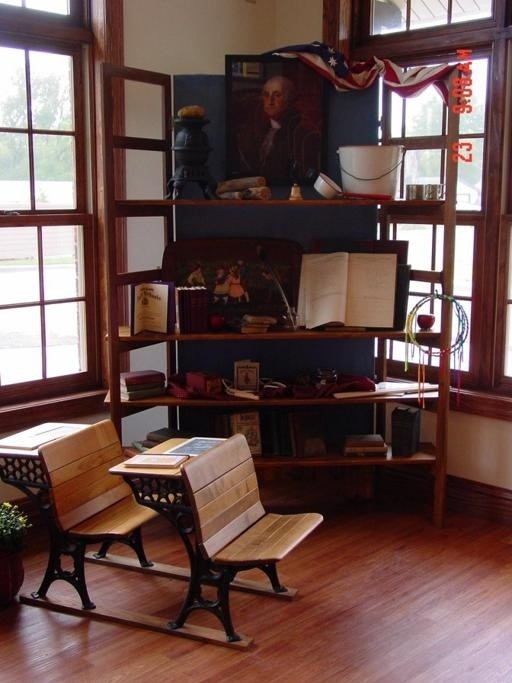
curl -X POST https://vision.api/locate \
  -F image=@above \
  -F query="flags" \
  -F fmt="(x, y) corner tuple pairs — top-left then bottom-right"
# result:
(272, 41), (461, 104)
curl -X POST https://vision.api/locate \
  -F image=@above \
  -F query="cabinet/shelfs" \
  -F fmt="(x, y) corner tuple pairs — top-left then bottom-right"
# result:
(99, 62), (469, 525)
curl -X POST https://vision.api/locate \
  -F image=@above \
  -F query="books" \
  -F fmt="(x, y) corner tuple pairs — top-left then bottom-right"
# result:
(176, 287), (209, 334)
(296, 252), (397, 330)
(344, 435), (388, 457)
(124, 454), (190, 469)
(164, 437), (227, 457)
(213, 407), (328, 457)
(234, 361), (259, 392)
(240, 314), (278, 334)
(120, 370), (166, 401)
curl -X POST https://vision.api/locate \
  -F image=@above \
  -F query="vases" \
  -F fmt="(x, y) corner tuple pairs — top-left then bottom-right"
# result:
(0, 544), (27, 605)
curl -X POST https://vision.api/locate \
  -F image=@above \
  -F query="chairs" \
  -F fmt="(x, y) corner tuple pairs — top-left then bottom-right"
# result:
(184, 434), (327, 641)
(38, 419), (166, 606)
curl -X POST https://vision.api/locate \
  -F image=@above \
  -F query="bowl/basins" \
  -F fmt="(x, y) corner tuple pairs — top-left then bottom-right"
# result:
(417, 314), (435, 329)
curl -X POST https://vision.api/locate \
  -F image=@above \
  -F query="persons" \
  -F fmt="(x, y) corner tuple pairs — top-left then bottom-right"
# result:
(236, 75), (321, 182)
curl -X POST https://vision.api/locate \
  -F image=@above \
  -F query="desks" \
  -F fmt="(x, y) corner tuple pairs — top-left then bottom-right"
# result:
(103, 437), (228, 632)
(2, 422), (92, 596)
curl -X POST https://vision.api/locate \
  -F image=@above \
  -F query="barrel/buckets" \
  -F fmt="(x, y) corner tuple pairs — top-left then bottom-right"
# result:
(336, 141), (406, 201)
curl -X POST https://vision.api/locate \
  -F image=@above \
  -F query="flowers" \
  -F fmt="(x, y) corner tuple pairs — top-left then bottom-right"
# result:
(0, 502), (33, 544)
